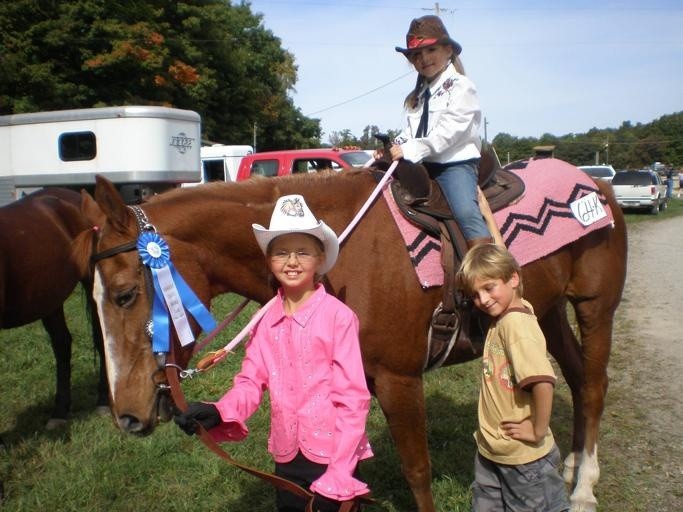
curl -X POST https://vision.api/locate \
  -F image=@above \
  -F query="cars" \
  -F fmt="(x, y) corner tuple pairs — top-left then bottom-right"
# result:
(575, 162), (666, 217)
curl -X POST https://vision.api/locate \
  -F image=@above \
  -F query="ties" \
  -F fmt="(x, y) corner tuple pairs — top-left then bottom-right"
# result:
(415, 89), (432, 140)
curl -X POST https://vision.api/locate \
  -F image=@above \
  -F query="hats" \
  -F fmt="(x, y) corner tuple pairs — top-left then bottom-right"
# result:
(252, 193), (340, 276)
(394, 15), (463, 61)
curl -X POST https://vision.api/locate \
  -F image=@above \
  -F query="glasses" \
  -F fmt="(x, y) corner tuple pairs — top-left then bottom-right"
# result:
(270, 246), (323, 264)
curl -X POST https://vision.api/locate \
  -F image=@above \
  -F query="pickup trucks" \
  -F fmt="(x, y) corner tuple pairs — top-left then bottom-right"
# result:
(233, 144), (371, 186)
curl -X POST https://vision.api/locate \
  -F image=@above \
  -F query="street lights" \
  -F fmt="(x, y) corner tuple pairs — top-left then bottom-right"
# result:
(603, 142), (611, 165)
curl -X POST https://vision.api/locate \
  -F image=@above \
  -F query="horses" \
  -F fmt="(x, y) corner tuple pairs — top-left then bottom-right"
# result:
(67, 158), (629, 512)
(0, 186), (112, 431)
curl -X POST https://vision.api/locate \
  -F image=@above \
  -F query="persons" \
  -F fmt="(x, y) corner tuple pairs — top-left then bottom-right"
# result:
(676, 168), (683, 198)
(663, 167), (675, 198)
(171, 193), (378, 510)
(372, 14), (496, 250)
(452, 182), (574, 511)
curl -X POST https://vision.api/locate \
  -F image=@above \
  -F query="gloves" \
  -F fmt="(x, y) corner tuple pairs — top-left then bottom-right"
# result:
(311, 492), (341, 511)
(175, 398), (219, 436)
